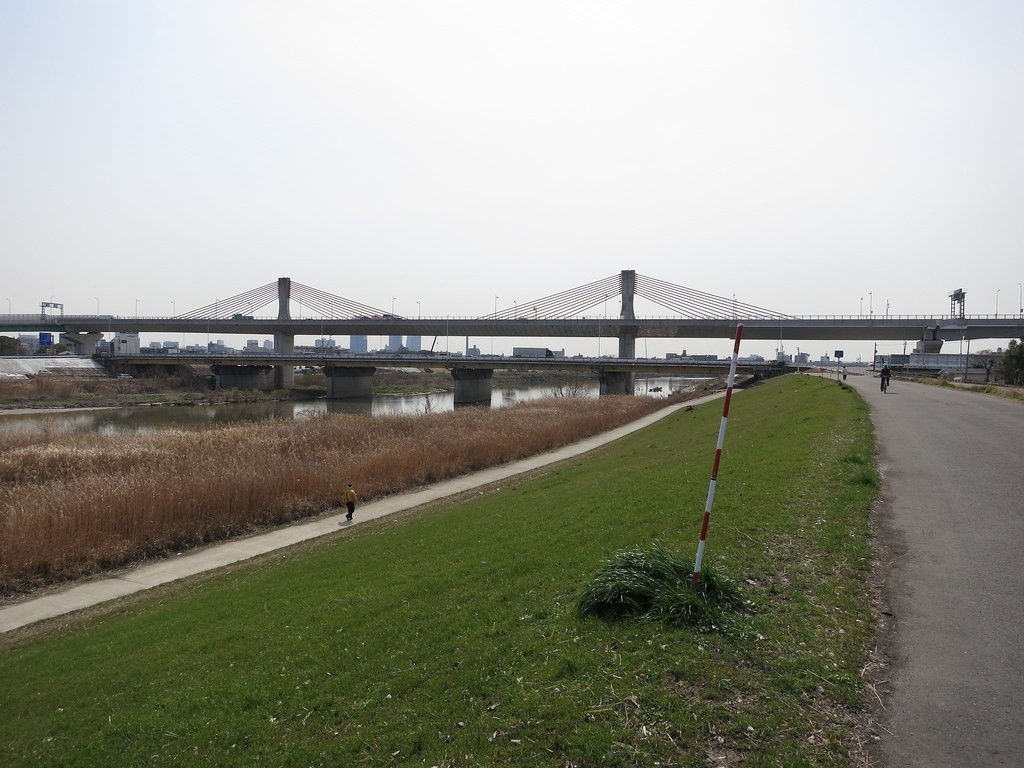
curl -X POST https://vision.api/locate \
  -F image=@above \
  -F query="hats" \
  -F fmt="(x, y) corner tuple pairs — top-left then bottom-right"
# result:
(347, 484), (353, 489)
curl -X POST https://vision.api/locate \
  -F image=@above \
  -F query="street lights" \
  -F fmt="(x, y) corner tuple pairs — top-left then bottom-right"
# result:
(299, 298), (303, 318)
(327, 300), (334, 320)
(869, 291), (873, 315)
(135, 297), (140, 317)
(215, 297), (220, 320)
(6, 297), (12, 315)
(1019, 282), (1022, 316)
(392, 295), (398, 319)
(995, 289), (1000, 315)
(604, 295), (609, 321)
(859, 298), (864, 317)
(170, 300), (176, 320)
(94, 297), (100, 316)
(50, 295), (54, 319)
(494, 295), (500, 320)
(416, 301), (422, 321)
(248, 301), (253, 318)
(533, 307), (539, 322)
(619, 300), (623, 311)
(732, 292), (737, 319)
(513, 299), (518, 320)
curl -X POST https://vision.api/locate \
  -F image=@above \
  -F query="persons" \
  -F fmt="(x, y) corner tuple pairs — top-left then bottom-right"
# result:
(342, 484), (358, 521)
(879, 365), (891, 391)
(842, 366), (847, 380)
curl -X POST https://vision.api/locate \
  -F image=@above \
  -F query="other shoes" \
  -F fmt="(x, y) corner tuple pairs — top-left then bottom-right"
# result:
(881, 390), (883, 391)
(350, 518), (353, 520)
(345, 515), (349, 521)
(887, 384), (889, 386)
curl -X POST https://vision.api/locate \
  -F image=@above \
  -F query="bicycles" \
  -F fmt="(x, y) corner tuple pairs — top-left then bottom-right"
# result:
(881, 374), (889, 393)
